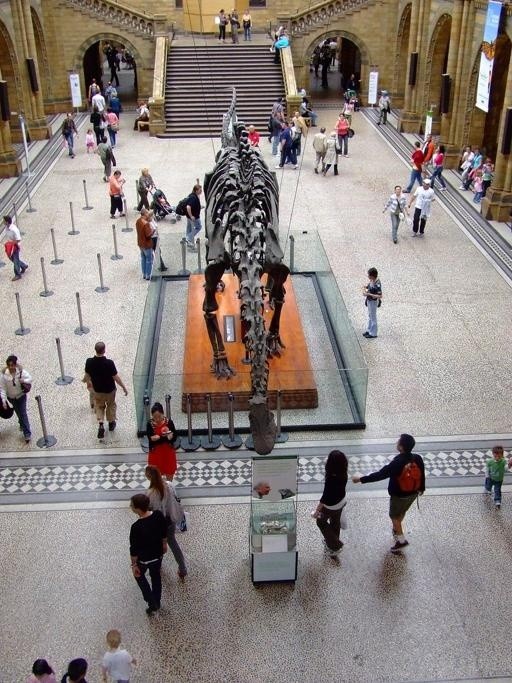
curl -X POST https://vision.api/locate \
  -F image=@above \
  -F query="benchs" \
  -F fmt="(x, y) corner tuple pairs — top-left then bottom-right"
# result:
(137, 120), (149, 132)
(303, 117), (312, 128)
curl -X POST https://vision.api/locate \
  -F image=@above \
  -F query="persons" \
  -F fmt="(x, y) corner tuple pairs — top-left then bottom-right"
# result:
(0, 356), (33, 441)
(311, 450), (348, 557)
(362, 268), (382, 337)
(484, 446), (512, 506)
(351, 434), (425, 551)
(61, 39), (202, 280)
(3, 216), (28, 281)
(101, 629), (136, 683)
(82, 342), (128, 438)
(129, 402), (188, 613)
(60, 658), (88, 683)
(28, 659), (57, 683)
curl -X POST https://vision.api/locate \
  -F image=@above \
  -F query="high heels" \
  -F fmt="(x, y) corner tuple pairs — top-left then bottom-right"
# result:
(177, 566), (188, 582)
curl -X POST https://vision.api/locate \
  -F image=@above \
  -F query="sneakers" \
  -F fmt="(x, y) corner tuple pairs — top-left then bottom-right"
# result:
(472, 198), (479, 203)
(314, 167), (339, 176)
(495, 499), (501, 505)
(437, 187), (447, 191)
(268, 137), (300, 170)
(339, 153), (350, 158)
(392, 237), (398, 244)
(18, 424), (24, 433)
(68, 153), (77, 159)
(459, 184), (468, 190)
(410, 231), (426, 237)
(109, 213), (126, 219)
(402, 188), (411, 193)
(97, 422), (105, 439)
(144, 602), (162, 618)
(11, 263), (29, 282)
(311, 123), (319, 128)
(327, 547), (344, 557)
(141, 275), (151, 281)
(108, 420), (117, 432)
(161, 267), (169, 272)
(388, 540), (410, 553)
(376, 121), (388, 126)
(110, 144), (117, 149)
(186, 238), (198, 253)
(487, 488), (493, 494)
(24, 434), (32, 442)
(102, 176), (111, 182)
(362, 331), (378, 339)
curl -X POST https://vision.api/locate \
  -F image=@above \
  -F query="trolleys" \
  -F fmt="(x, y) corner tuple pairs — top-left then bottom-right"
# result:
(330, 126), (341, 153)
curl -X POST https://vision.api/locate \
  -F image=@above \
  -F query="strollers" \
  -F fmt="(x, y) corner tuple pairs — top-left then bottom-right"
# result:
(148, 185), (183, 224)
(344, 89), (360, 112)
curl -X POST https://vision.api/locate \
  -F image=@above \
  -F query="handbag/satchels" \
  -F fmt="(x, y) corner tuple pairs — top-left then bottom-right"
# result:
(19, 370), (31, 394)
(398, 211), (406, 222)
(91, 84), (97, 96)
(0, 396), (15, 420)
(164, 481), (185, 524)
(266, 115), (275, 133)
(467, 166), (476, 176)
(345, 118), (355, 138)
(139, 107), (148, 121)
(365, 297), (383, 308)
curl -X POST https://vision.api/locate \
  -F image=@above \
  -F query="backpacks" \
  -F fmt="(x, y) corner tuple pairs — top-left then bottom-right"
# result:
(107, 87), (115, 96)
(398, 460), (422, 492)
